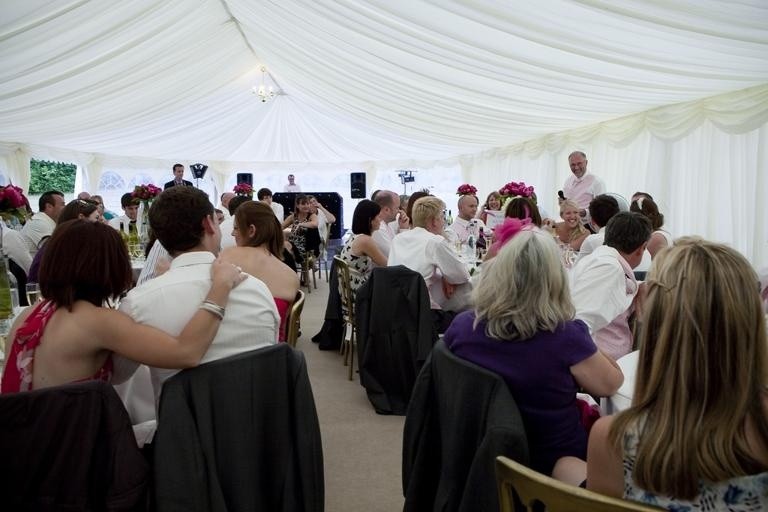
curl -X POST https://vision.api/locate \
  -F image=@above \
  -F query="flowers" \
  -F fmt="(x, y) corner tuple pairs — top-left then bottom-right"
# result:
(499, 180), (535, 197)
(129, 183), (162, 200)
(0, 184), (32, 223)
(233, 183), (254, 194)
(457, 183), (477, 197)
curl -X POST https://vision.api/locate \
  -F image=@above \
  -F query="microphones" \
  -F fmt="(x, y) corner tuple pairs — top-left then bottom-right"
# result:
(558, 191), (565, 200)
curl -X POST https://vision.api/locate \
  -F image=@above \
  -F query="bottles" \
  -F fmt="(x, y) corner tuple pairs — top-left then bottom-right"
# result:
(0, 223), (16, 362)
(447, 209), (486, 267)
(118, 220), (150, 258)
(3, 253), (20, 318)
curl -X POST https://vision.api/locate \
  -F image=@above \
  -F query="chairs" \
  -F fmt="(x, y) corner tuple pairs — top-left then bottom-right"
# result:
(356, 265), (446, 416)
(288, 224), (358, 380)
(495, 456), (662, 512)
(140, 341), (324, 511)
(401, 337), (528, 511)
(1, 379), (156, 511)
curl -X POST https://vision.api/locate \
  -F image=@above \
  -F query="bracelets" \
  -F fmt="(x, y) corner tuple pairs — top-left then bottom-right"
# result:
(198, 298), (227, 321)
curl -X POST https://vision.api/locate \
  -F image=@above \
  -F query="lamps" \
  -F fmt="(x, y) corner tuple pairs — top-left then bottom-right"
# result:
(252, 67), (273, 103)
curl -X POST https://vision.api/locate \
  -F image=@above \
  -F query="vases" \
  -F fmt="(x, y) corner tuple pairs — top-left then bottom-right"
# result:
(141, 200), (151, 235)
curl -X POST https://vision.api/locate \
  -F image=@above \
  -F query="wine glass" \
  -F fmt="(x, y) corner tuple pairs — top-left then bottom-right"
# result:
(26, 282), (44, 306)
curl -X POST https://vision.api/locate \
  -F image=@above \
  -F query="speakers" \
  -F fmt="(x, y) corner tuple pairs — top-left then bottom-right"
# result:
(351, 172), (366, 198)
(237, 173), (253, 200)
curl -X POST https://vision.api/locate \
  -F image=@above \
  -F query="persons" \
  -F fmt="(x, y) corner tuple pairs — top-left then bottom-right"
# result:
(107, 186), (283, 448)
(217, 200), (301, 342)
(0, 218), (249, 393)
(550, 233), (768, 512)
(1, 191), (108, 308)
(135, 196), (174, 287)
(565, 210), (649, 361)
(339, 189), (676, 334)
(257, 187), (335, 286)
(108, 193), (143, 234)
(556, 152), (603, 235)
(283, 174), (301, 192)
(216, 193), (251, 253)
(443, 229), (623, 476)
(164, 164), (192, 190)
